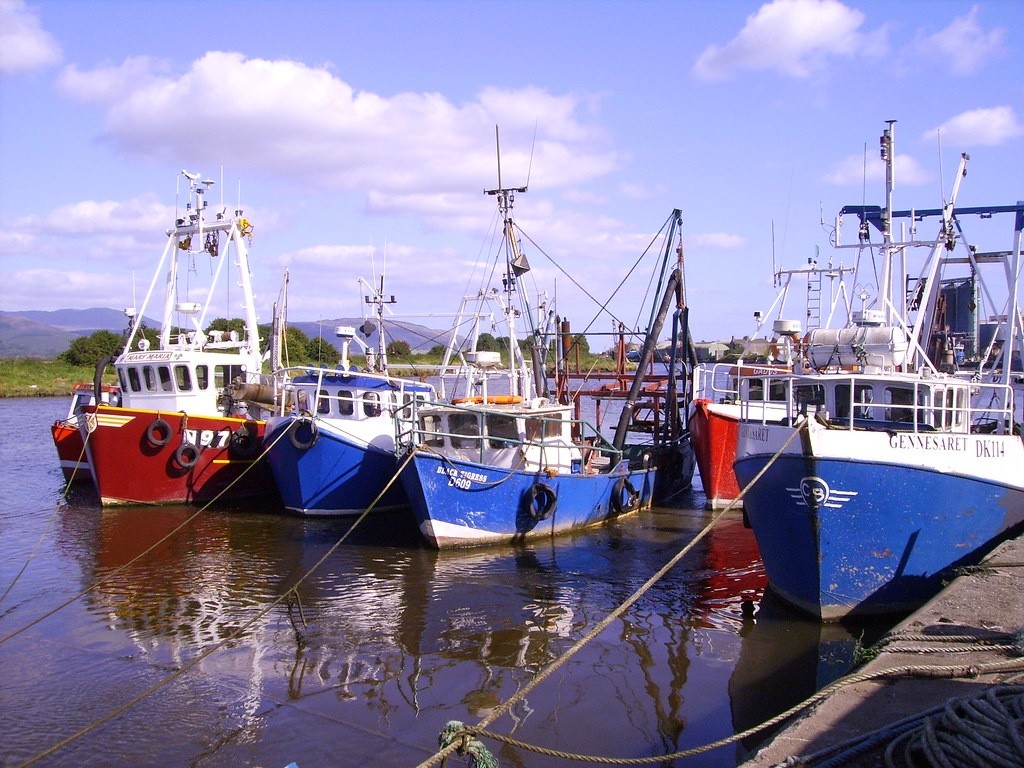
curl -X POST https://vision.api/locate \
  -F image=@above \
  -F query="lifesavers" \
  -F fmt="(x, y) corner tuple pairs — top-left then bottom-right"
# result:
(523, 483), (557, 520)
(287, 418), (319, 452)
(229, 428), (259, 458)
(487, 395), (523, 403)
(769, 333), (801, 361)
(612, 478), (637, 512)
(803, 328), (818, 358)
(450, 397), (483, 405)
(146, 418), (173, 446)
(175, 443), (199, 469)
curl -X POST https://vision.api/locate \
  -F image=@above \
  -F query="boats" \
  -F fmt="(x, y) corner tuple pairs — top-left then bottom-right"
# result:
(51, 118), (1023, 628)
